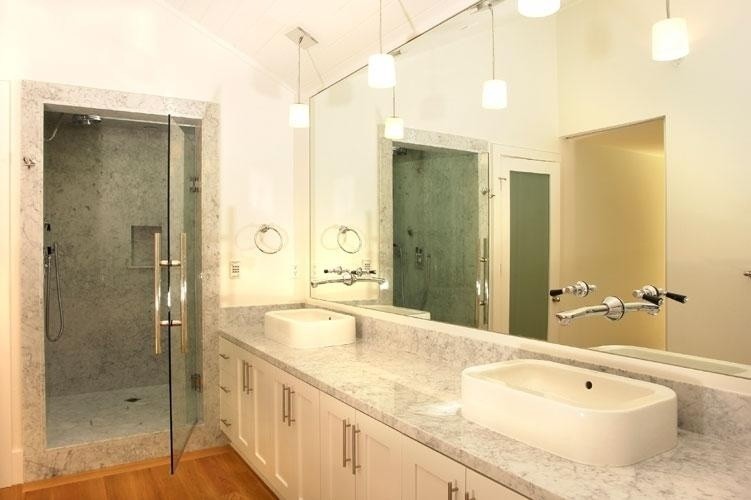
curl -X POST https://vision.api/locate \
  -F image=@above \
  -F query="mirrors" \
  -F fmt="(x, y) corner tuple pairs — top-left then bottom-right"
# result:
(308, 0), (751, 382)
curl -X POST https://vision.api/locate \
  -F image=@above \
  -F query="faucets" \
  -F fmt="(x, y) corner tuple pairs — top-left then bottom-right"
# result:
(310, 277), (345, 286)
(353, 277), (385, 285)
(554, 304), (608, 326)
(624, 301), (661, 316)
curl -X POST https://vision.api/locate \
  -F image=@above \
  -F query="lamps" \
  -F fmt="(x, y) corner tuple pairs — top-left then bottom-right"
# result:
(384, 48), (404, 140)
(367, 0), (397, 90)
(652, 0), (687, 61)
(480, 3), (507, 111)
(516, 0), (560, 17)
(280, 27), (316, 131)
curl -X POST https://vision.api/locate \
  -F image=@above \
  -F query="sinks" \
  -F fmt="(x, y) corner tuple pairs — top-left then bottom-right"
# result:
(460, 360), (678, 466)
(590, 343), (750, 380)
(263, 308), (356, 348)
(360, 304), (431, 319)
